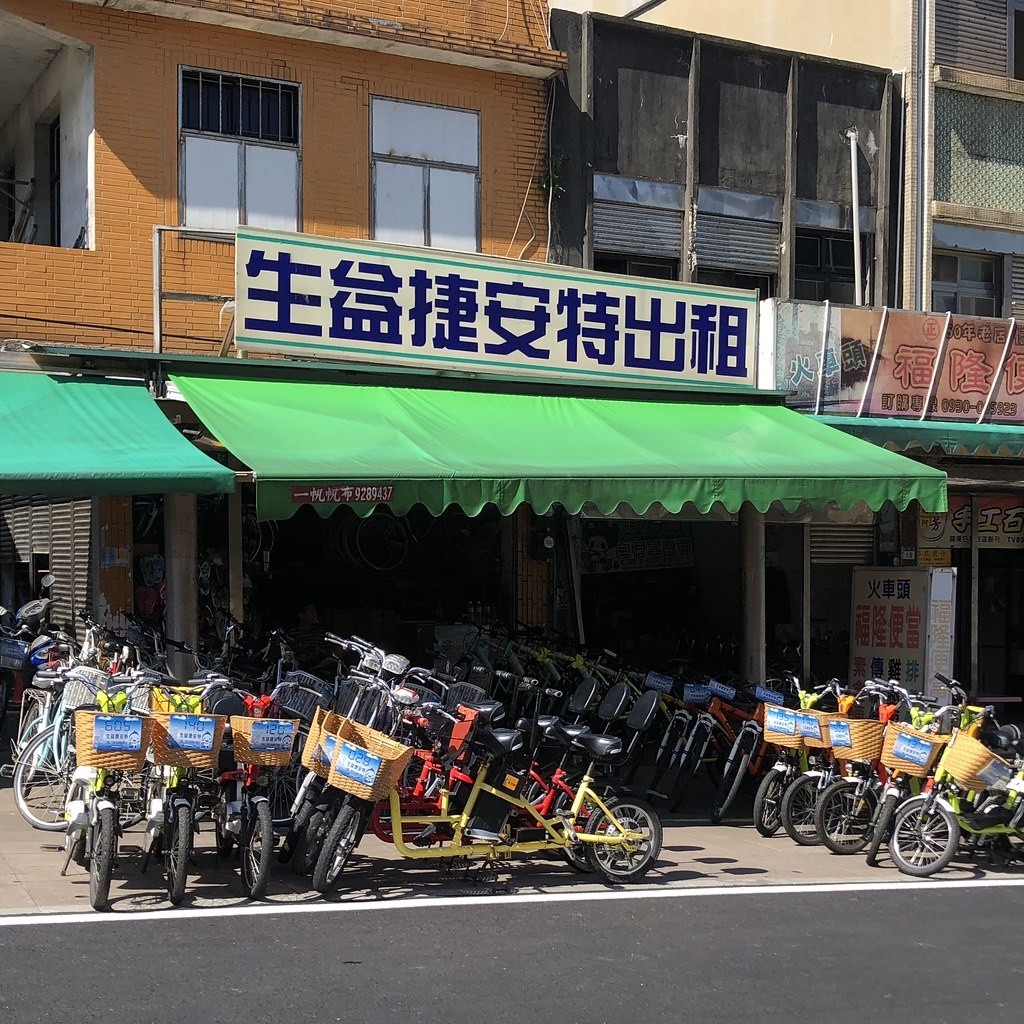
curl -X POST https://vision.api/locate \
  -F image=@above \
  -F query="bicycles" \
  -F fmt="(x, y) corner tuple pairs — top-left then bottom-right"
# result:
(0, 596), (1024, 911)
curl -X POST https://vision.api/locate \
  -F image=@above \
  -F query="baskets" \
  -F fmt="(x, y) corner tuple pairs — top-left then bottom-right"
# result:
(880, 719), (951, 778)
(63, 666), (107, 712)
(444, 681), (485, 713)
(308, 710), (345, 777)
(828, 718), (885, 761)
(764, 702), (804, 748)
(335, 680), (377, 724)
(302, 705), (328, 766)
(0, 636), (30, 670)
(74, 710), (150, 772)
(149, 712), (228, 768)
(796, 708), (848, 748)
(281, 669), (333, 723)
(230, 715), (300, 765)
(943, 731), (1010, 795)
(327, 718), (415, 802)
(126, 685), (150, 715)
(392, 683), (441, 731)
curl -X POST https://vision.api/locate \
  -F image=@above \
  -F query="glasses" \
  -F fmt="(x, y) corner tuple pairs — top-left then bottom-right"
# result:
(49, 644), (60, 655)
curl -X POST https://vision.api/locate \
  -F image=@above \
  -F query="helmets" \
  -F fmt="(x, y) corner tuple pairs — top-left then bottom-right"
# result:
(27, 635), (57, 667)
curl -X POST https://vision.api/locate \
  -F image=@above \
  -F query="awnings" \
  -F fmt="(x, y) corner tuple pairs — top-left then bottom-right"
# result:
(1, 362), (1024, 525)
